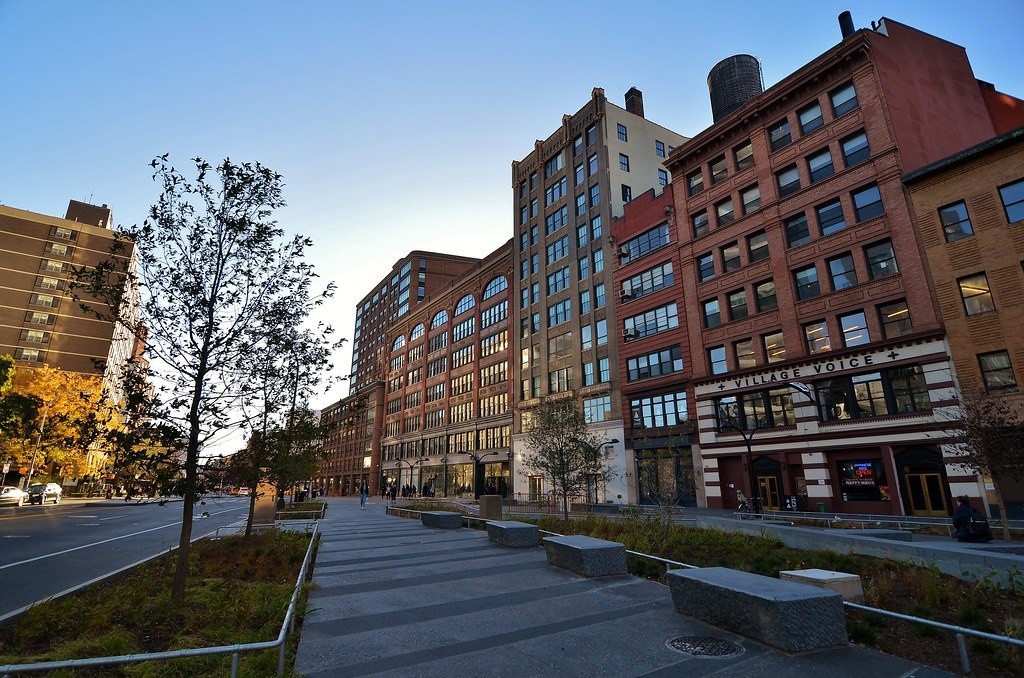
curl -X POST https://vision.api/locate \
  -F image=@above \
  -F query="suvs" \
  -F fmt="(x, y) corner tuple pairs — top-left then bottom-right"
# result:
(24, 483), (62, 505)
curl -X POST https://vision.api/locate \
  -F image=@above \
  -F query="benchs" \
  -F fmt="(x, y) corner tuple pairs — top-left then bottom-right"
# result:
(486, 521), (539, 547)
(420, 511), (462, 529)
(667, 567), (848, 651)
(542, 535), (627, 576)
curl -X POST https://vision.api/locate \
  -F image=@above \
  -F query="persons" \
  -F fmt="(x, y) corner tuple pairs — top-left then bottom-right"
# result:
(381, 482), (437, 502)
(360, 479), (370, 509)
(484, 483), (496, 495)
(460, 483), (472, 497)
(952, 495), (993, 544)
(302, 485), (326, 498)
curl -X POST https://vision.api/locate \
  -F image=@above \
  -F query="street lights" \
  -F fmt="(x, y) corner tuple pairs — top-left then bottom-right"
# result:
(456, 450), (499, 500)
(719, 418), (771, 513)
(568, 437), (619, 504)
(394, 456), (430, 499)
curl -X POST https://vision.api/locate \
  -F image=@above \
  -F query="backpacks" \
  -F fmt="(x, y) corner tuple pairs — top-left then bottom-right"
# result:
(966, 510), (994, 543)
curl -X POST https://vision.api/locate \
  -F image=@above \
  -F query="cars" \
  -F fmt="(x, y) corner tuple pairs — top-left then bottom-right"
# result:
(231, 487), (250, 497)
(0, 486), (31, 507)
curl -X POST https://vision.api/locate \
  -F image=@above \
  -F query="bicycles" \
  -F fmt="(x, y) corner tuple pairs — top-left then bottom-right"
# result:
(735, 489), (766, 519)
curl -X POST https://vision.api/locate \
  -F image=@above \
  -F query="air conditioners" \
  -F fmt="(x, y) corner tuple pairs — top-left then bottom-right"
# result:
(616, 247), (627, 255)
(619, 289), (631, 297)
(622, 328), (634, 336)
